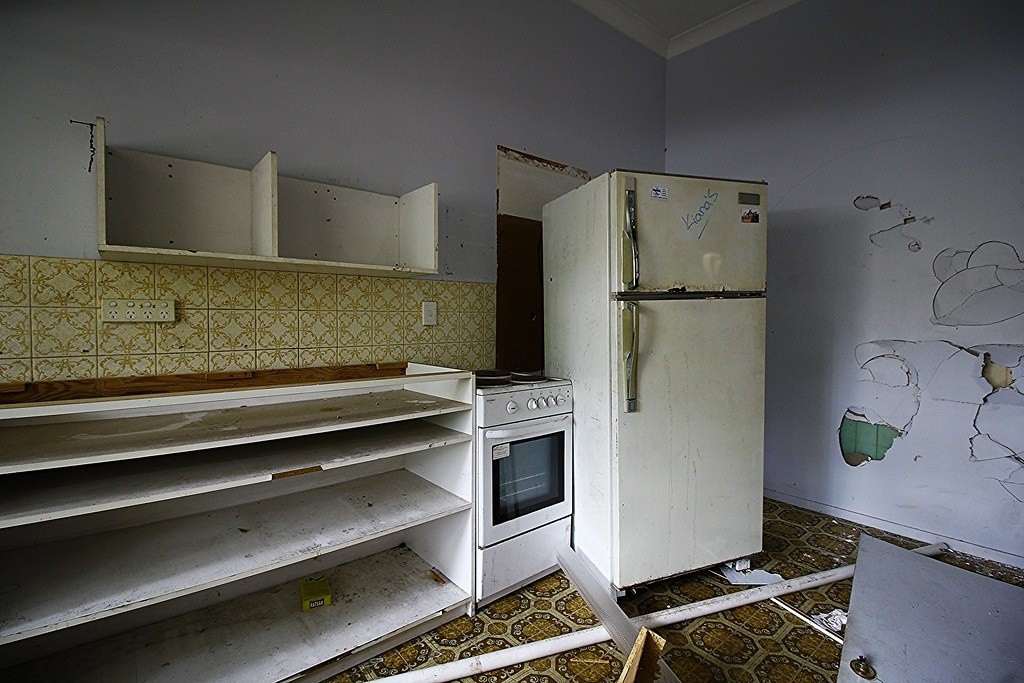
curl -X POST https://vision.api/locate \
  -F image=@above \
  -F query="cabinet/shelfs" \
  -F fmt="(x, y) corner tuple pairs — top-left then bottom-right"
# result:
(0, 360), (476, 683)
(96, 116), (438, 277)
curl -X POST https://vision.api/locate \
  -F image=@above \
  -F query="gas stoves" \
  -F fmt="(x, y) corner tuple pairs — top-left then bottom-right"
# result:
(473, 368), (573, 427)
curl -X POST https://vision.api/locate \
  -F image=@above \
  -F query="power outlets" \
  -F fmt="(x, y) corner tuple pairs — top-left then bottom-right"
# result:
(100, 299), (176, 322)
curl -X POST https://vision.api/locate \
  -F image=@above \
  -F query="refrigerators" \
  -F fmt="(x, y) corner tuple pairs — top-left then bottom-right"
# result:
(542, 167), (767, 587)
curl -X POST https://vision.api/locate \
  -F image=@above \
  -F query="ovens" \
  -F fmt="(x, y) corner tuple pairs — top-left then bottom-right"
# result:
(476, 413), (575, 610)
